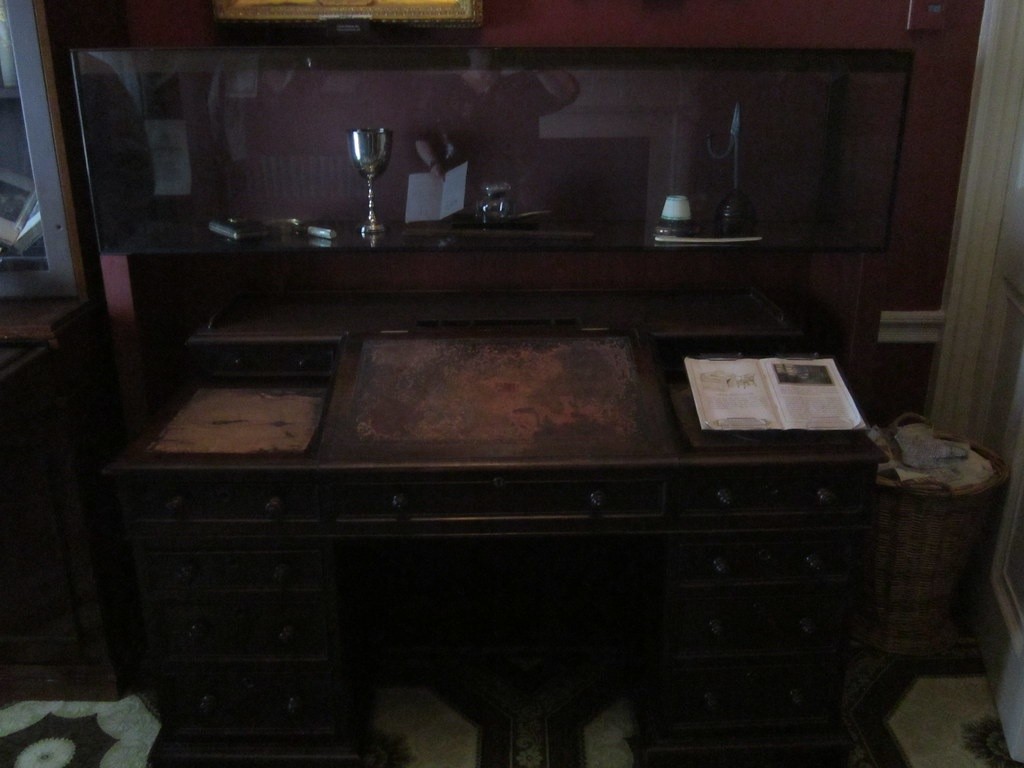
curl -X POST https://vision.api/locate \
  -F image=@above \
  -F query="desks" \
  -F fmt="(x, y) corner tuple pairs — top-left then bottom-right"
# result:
(101, 367), (891, 768)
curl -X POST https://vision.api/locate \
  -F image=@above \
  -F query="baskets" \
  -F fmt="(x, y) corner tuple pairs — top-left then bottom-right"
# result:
(846, 412), (1014, 660)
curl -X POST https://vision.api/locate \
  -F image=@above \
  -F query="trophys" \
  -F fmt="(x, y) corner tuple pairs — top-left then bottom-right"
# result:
(345, 129), (394, 236)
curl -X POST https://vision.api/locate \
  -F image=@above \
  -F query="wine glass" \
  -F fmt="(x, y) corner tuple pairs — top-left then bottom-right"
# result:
(346, 128), (394, 235)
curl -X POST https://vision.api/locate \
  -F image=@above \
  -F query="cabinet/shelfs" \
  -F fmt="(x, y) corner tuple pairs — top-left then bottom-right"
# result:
(99, 217), (807, 378)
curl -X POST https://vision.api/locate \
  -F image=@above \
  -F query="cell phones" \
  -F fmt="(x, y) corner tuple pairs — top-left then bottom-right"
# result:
(208, 217), (269, 242)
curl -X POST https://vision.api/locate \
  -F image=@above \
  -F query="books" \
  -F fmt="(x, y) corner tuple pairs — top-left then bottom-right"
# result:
(683, 356), (869, 432)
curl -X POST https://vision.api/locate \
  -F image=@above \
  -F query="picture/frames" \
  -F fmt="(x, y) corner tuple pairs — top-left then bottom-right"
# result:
(209, 0), (483, 33)
